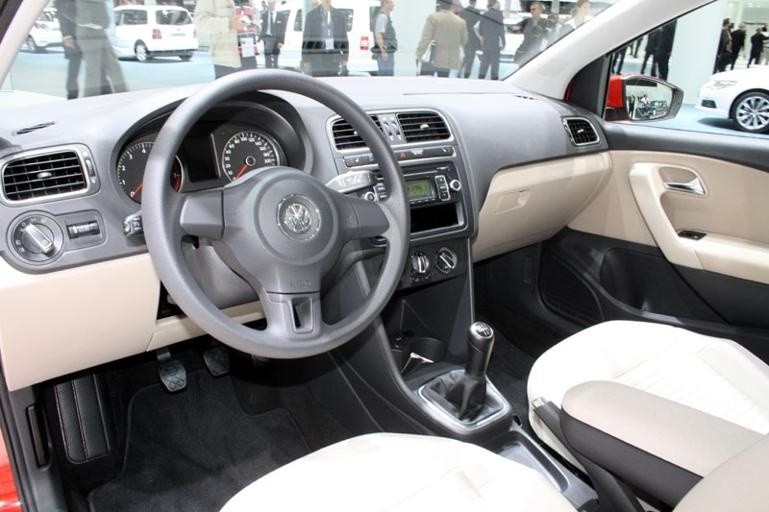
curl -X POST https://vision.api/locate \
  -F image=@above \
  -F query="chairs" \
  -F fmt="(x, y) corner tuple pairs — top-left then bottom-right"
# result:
(219, 431), (764, 511)
(527, 318), (763, 507)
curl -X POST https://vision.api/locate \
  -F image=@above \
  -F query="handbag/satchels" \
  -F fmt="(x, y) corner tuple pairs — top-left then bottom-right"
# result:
(422, 40), (436, 64)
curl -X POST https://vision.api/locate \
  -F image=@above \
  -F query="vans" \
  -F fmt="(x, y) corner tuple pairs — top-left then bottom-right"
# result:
(104, 4), (199, 63)
(250, 2), (389, 77)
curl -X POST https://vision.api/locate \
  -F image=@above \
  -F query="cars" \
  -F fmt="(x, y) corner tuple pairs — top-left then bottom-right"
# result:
(461, 8), (585, 61)
(647, 100), (667, 118)
(694, 65), (769, 133)
(22, 7), (66, 54)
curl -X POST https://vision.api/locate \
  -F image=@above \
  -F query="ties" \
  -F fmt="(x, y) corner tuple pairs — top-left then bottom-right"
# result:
(270, 12), (274, 35)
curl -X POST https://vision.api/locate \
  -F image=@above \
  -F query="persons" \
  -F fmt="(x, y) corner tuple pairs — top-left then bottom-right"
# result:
(514, 0), (590, 63)
(640, 94), (649, 106)
(713, 18), (768, 72)
(56, 1), (129, 99)
(192, 0), (286, 79)
(301, 1), (349, 78)
(374, 1), (397, 76)
(627, 92), (636, 119)
(612, 20), (676, 81)
(415, 1), (506, 80)
